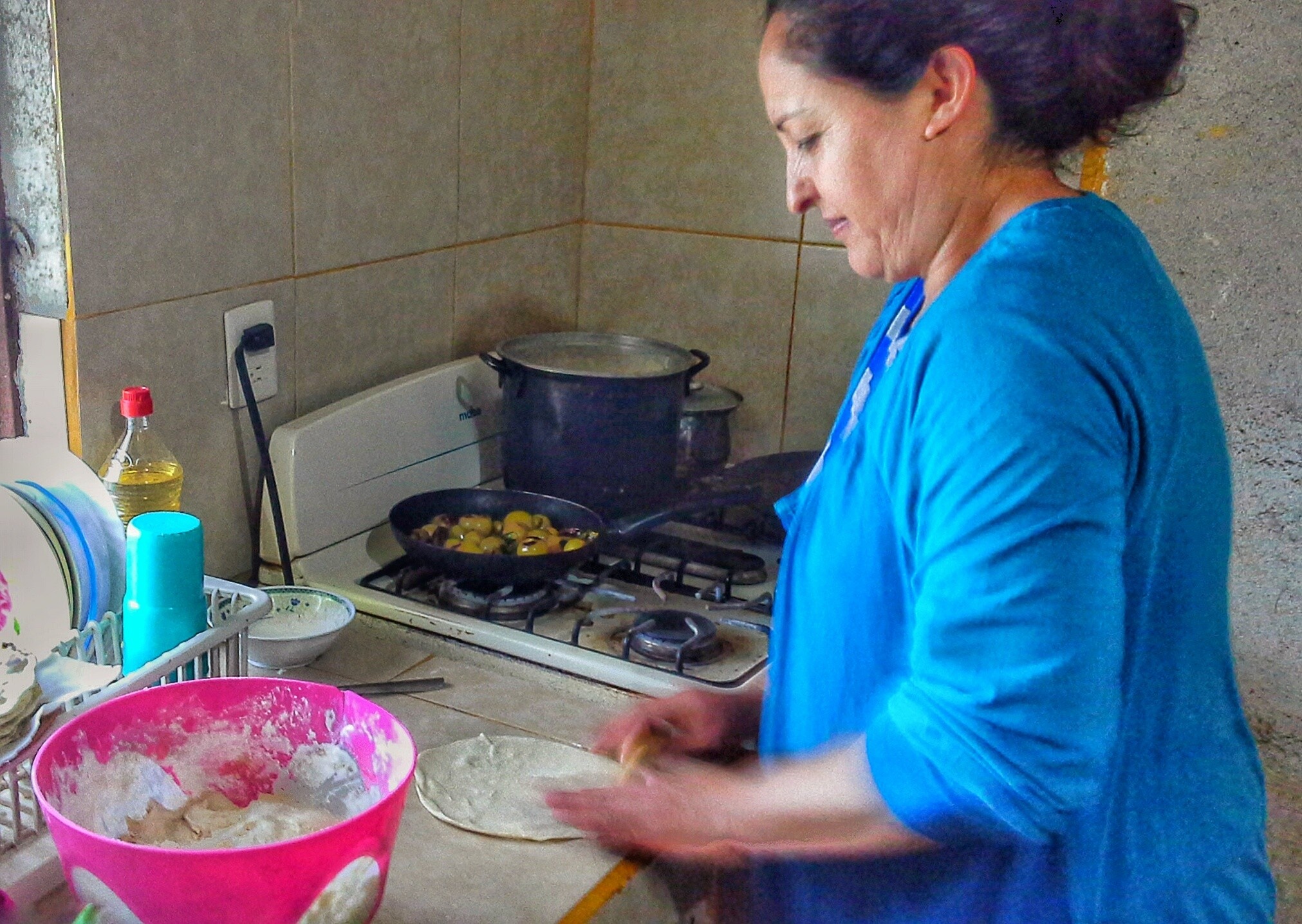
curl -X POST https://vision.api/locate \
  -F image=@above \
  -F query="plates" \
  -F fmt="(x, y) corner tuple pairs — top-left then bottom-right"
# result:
(0, 436), (126, 662)
(0, 639), (121, 766)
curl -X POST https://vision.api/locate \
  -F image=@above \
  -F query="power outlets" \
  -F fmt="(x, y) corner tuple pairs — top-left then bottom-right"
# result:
(224, 300), (278, 409)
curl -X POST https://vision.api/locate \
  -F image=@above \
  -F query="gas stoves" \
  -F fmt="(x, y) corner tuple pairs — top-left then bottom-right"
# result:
(258, 350), (782, 701)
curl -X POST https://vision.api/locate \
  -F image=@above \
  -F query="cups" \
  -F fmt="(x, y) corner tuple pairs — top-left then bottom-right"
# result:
(122, 511), (208, 689)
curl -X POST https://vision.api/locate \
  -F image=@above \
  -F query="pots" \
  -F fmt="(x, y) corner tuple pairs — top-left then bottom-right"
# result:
(388, 487), (730, 586)
(677, 376), (743, 477)
(478, 332), (712, 525)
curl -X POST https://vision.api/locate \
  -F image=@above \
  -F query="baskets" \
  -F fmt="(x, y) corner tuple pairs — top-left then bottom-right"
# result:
(1, 575), (272, 860)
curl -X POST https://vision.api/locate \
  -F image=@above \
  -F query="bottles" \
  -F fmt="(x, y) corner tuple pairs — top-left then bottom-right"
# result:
(99, 386), (183, 538)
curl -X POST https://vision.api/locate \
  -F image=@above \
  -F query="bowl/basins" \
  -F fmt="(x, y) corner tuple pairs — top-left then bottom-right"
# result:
(15, 479), (111, 653)
(30, 676), (417, 924)
(206, 585), (355, 669)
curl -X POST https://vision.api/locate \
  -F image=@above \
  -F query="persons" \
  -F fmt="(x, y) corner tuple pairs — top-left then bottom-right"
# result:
(546, 0), (1279, 924)
